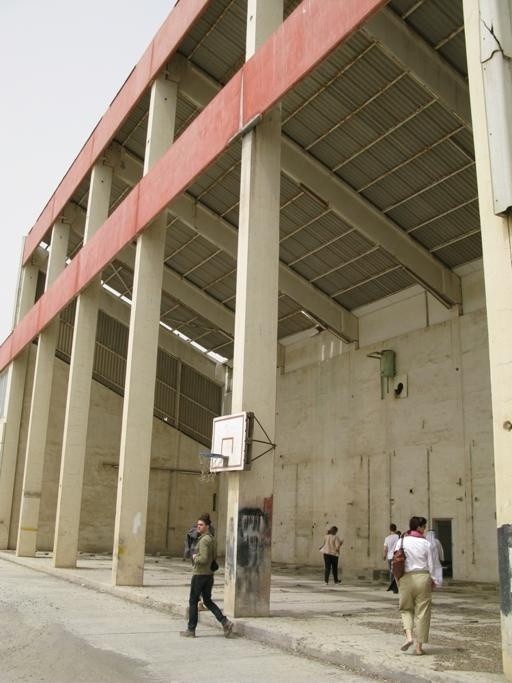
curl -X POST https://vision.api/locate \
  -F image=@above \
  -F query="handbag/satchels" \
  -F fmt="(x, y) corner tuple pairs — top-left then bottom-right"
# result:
(393, 537), (406, 581)
(210, 560), (219, 571)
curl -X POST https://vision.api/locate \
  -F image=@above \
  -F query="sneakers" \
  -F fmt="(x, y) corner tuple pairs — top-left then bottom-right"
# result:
(180, 629), (195, 638)
(400, 640), (425, 655)
(198, 600), (209, 612)
(222, 618), (234, 638)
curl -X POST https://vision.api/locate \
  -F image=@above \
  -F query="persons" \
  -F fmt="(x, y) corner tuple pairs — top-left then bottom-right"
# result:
(319, 526), (343, 585)
(180, 513), (234, 638)
(383, 516), (444, 655)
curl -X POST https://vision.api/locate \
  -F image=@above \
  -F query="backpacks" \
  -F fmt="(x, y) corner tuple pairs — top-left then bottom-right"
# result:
(184, 527), (201, 567)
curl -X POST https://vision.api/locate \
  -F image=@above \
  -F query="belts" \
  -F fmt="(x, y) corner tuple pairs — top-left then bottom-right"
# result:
(408, 571), (429, 574)
